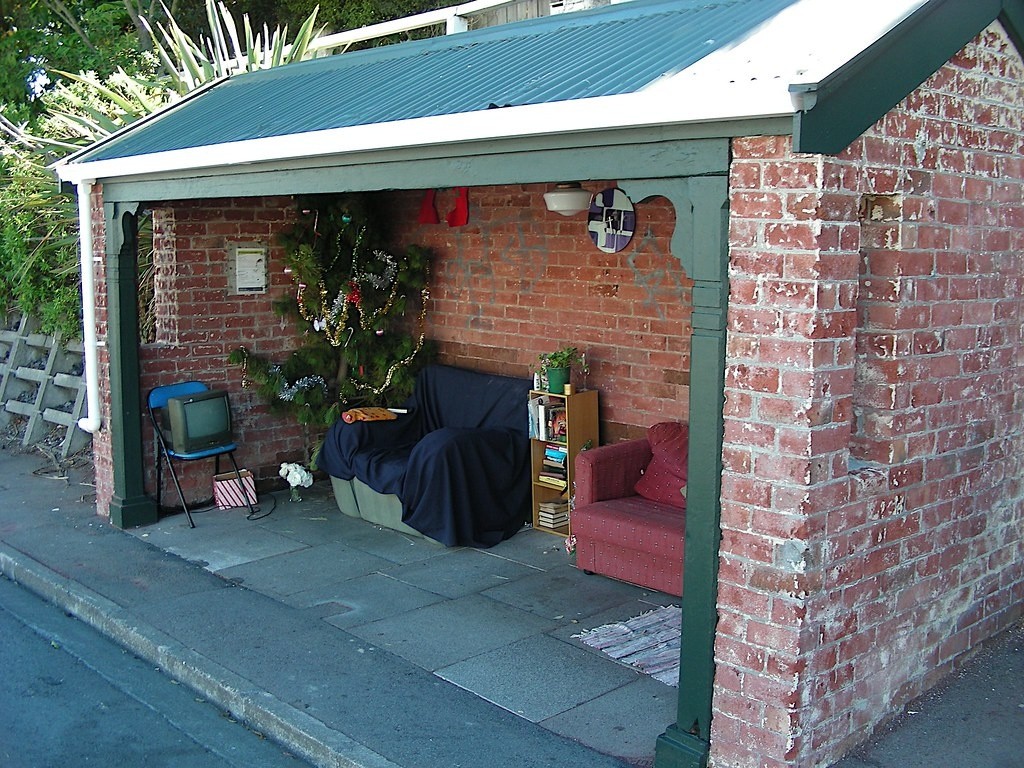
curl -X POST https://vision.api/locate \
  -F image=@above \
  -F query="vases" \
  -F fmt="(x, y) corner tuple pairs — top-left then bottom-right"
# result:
(290, 485), (303, 502)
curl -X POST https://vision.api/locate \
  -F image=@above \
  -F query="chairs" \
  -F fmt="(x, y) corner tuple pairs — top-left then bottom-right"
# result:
(149, 381), (254, 529)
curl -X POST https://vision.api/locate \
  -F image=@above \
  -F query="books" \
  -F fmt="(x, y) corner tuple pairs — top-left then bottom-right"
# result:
(528, 395), (569, 530)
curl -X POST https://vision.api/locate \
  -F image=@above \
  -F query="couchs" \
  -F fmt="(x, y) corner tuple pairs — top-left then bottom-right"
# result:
(316, 364), (534, 550)
(571, 422), (689, 597)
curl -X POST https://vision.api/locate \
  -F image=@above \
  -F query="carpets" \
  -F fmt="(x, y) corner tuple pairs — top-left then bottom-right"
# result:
(570, 604), (681, 688)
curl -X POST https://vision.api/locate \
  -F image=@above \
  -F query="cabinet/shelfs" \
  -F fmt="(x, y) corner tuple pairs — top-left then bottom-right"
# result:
(530, 390), (599, 536)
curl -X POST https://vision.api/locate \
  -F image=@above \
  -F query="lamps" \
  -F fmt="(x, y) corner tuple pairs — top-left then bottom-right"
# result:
(543, 181), (592, 217)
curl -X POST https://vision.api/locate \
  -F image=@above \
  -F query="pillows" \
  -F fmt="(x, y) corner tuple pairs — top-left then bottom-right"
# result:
(634, 421), (687, 508)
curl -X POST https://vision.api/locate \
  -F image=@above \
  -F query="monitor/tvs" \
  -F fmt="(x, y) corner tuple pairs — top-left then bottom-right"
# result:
(160, 390), (233, 454)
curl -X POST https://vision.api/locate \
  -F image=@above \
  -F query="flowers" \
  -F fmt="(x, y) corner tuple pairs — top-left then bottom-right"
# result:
(279, 462), (314, 499)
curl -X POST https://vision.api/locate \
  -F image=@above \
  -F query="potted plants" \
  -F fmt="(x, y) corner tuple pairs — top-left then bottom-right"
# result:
(529, 347), (591, 394)
